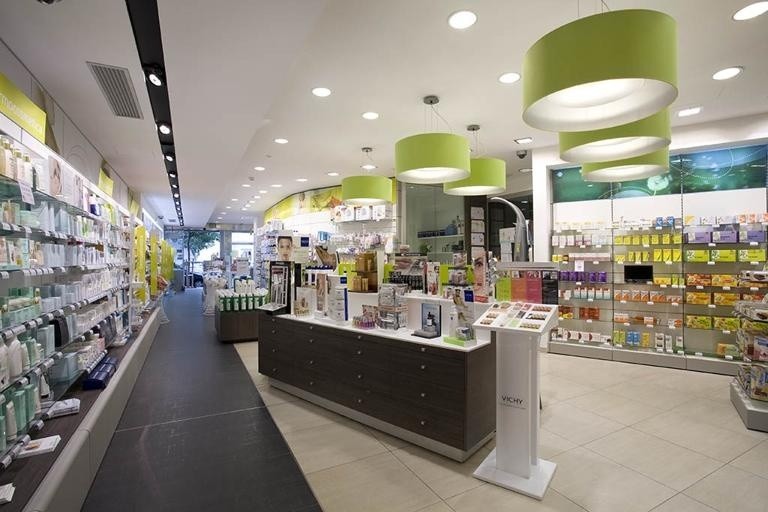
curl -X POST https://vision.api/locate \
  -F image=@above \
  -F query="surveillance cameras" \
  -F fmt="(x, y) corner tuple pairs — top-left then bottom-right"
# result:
(517, 152), (529, 159)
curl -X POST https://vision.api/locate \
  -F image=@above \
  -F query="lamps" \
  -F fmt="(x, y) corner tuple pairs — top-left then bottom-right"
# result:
(554, 103), (674, 170)
(515, 5), (678, 133)
(394, 131), (473, 188)
(578, 144), (671, 186)
(339, 167), (396, 211)
(440, 153), (510, 198)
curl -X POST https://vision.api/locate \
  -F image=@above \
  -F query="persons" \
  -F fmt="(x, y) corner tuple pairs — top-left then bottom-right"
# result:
(430, 266), (439, 293)
(277, 235), (293, 260)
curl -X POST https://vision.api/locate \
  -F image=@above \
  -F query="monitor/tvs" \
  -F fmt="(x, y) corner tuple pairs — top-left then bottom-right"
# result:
(427, 252), (454, 266)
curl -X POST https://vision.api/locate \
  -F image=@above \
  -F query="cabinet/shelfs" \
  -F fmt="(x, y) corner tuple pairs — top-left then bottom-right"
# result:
(539, 210), (768, 435)
(0, 118), (170, 512)
(209, 218), (564, 500)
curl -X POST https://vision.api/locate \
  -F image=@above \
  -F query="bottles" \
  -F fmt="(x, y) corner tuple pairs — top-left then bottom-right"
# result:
(455, 216), (461, 235)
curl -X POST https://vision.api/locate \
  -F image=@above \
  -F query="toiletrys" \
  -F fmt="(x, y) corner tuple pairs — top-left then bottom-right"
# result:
(216, 280), (269, 311)
(0, 136), (131, 459)
